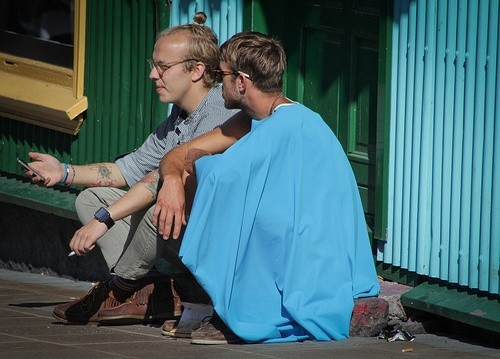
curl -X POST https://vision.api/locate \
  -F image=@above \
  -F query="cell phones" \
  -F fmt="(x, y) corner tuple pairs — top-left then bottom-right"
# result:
(16, 157), (46, 181)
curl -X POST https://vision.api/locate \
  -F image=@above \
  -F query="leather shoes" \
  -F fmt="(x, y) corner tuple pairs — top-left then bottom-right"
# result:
(52, 280), (123, 324)
(97, 276), (182, 325)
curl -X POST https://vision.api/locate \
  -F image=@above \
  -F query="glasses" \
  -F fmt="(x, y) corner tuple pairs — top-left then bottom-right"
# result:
(148, 58), (199, 75)
(213, 70), (253, 83)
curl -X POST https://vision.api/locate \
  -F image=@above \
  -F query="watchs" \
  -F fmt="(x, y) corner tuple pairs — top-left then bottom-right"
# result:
(93, 207), (115, 230)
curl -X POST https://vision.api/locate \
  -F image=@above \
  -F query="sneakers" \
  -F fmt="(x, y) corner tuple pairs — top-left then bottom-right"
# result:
(191, 309), (243, 344)
(161, 319), (191, 337)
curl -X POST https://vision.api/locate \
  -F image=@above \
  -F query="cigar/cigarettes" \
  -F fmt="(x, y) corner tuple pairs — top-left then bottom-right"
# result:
(238, 71), (253, 81)
(69, 251), (75, 256)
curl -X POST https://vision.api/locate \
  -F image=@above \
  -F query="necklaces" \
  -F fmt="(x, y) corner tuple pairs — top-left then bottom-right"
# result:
(268, 92), (282, 116)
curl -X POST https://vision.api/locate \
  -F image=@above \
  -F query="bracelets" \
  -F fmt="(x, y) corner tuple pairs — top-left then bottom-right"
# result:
(61, 163), (69, 184)
(66, 164), (76, 186)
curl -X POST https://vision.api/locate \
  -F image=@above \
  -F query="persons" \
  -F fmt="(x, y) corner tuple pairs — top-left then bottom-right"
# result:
(153, 32), (378, 345)
(23, 23), (242, 325)
